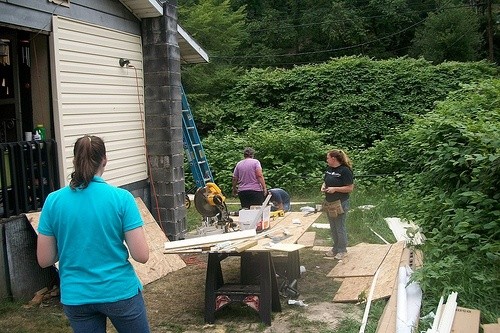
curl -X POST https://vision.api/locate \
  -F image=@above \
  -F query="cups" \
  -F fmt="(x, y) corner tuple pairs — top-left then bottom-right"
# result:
(25, 132), (32, 141)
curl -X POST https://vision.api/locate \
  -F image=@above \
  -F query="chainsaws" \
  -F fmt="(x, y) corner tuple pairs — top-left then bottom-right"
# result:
(194, 181), (234, 235)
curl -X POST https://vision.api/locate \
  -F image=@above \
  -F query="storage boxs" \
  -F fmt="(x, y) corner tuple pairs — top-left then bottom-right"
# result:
(238, 205), (271, 231)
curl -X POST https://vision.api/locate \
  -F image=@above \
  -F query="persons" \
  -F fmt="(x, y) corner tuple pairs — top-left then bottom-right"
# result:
(232, 148), (267, 210)
(265, 188), (291, 213)
(321, 149), (354, 260)
(38, 136), (149, 333)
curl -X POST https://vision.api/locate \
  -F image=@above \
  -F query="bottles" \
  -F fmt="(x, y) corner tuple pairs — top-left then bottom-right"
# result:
(32, 125), (47, 140)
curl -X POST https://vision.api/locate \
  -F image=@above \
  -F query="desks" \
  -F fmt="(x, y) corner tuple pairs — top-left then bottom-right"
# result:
(163, 211), (323, 326)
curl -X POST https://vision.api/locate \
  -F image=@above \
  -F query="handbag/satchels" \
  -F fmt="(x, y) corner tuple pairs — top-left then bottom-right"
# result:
(322, 197), (344, 218)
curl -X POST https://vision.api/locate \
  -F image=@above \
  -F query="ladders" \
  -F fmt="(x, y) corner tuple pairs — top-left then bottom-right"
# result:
(180, 79), (214, 206)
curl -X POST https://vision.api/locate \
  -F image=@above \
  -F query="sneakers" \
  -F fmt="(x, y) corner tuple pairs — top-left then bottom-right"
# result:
(334, 252), (348, 260)
(324, 250), (335, 257)
